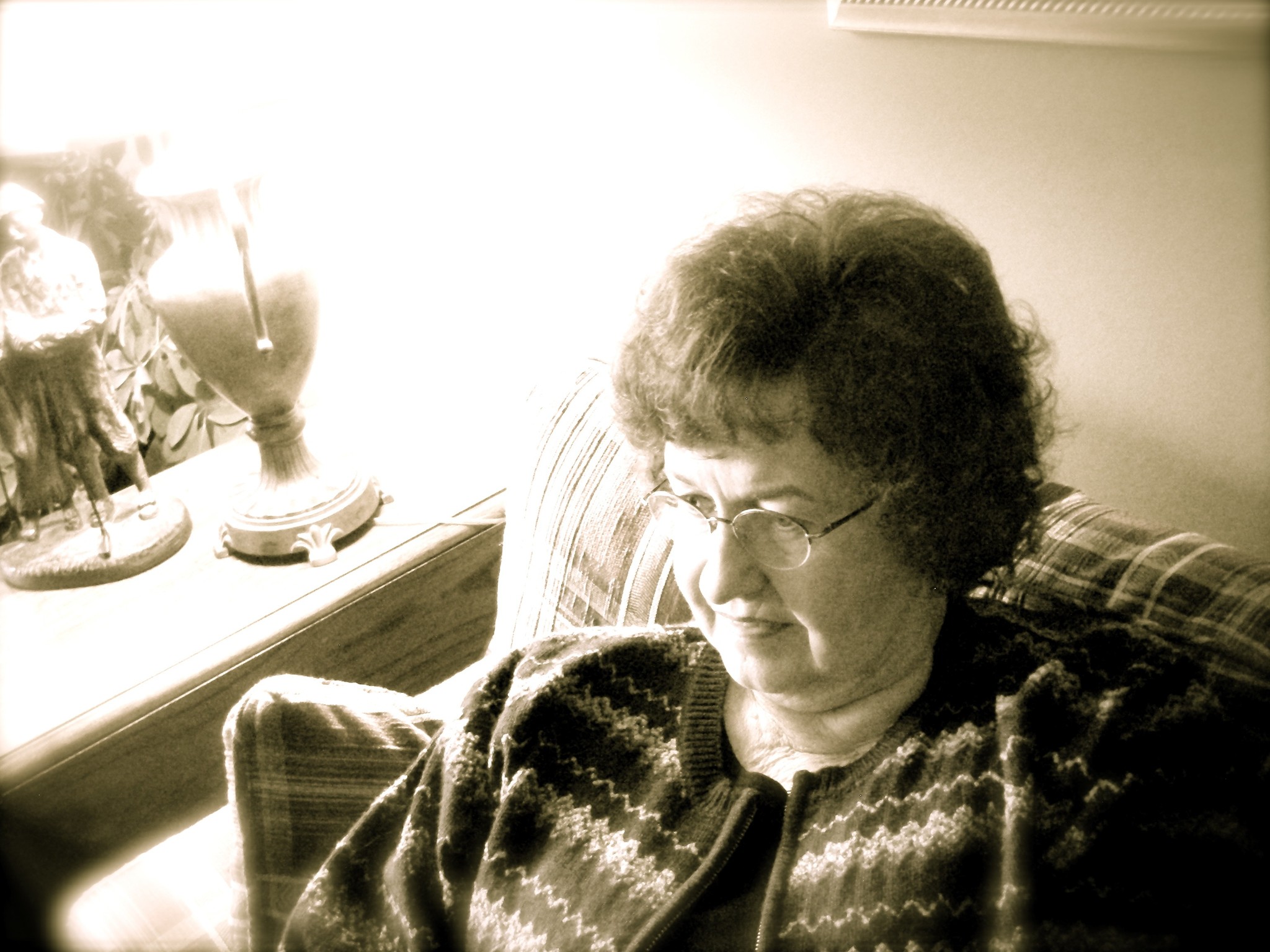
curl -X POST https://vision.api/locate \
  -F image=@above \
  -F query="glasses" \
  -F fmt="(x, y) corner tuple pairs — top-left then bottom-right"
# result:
(640, 480), (887, 569)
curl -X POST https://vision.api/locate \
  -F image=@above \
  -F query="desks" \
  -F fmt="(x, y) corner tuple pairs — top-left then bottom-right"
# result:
(0, 453), (510, 847)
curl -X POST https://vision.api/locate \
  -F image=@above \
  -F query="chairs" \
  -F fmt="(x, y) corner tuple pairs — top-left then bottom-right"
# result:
(55, 336), (1270, 952)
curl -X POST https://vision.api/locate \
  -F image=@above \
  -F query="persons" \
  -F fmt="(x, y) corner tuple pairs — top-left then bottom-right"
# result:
(271, 180), (1270, 951)
(0, 182), (154, 527)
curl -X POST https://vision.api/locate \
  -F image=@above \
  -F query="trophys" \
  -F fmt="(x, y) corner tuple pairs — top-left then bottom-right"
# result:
(141, 210), (383, 562)
